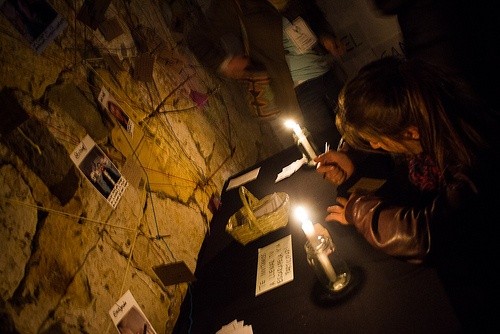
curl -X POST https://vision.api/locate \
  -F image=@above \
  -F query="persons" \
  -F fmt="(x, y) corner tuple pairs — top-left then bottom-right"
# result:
(108, 101), (129, 130)
(188, 1), (350, 152)
(88, 162), (120, 199)
(315, 57), (500, 304)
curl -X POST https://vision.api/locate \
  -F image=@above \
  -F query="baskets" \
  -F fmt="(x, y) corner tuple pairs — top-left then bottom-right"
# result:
(224, 186), (290, 247)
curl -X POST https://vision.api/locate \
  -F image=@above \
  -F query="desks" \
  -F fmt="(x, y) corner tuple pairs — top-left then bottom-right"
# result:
(171, 126), (471, 333)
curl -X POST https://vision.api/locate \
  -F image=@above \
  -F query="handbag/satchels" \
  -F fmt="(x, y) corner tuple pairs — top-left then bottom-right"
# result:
(242, 68), (282, 123)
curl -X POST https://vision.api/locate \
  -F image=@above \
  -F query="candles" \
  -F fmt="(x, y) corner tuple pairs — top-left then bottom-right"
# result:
(285, 119), (318, 162)
(294, 208), (338, 281)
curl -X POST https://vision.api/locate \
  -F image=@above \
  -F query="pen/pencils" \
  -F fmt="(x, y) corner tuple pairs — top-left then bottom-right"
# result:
(321, 141), (331, 180)
